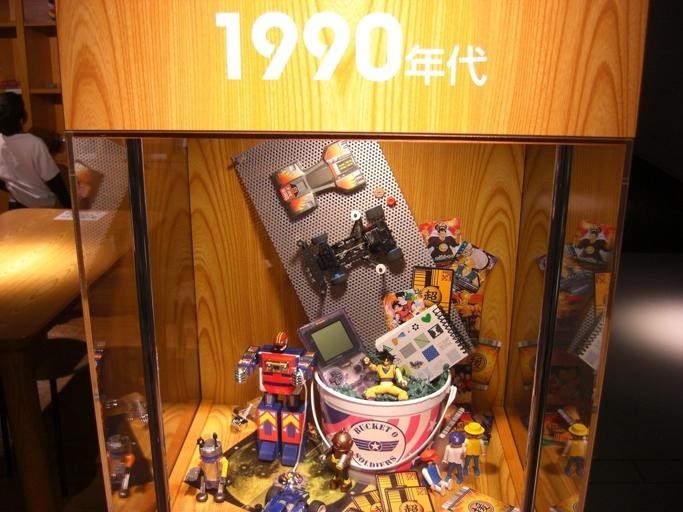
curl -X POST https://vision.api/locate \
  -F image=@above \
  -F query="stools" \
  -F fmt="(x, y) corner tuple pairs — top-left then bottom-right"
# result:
(35, 338), (113, 499)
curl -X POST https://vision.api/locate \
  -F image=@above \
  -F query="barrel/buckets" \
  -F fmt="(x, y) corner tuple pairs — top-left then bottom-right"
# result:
(310, 370), (458, 474)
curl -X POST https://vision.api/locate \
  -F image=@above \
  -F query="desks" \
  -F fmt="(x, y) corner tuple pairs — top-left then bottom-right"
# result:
(0, 207), (164, 512)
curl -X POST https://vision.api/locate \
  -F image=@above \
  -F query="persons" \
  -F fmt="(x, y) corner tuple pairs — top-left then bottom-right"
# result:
(0, 91), (71, 211)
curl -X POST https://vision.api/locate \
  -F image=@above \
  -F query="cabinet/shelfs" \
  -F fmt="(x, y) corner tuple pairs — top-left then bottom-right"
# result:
(1, 0), (68, 163)
